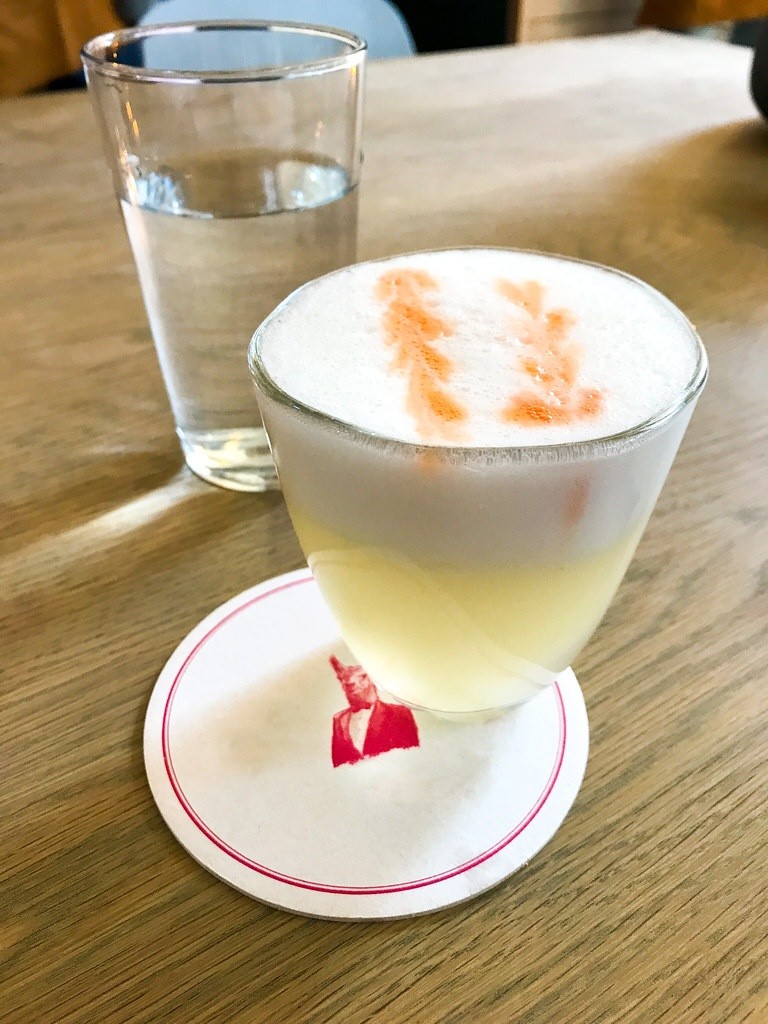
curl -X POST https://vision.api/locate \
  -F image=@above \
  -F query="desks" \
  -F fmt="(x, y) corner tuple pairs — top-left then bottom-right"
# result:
(0, 26), (768, 1024)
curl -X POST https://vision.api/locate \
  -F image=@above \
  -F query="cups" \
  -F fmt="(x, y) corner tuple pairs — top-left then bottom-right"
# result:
(248, 245), (708, 714)
(79, 18), (366, 493)
